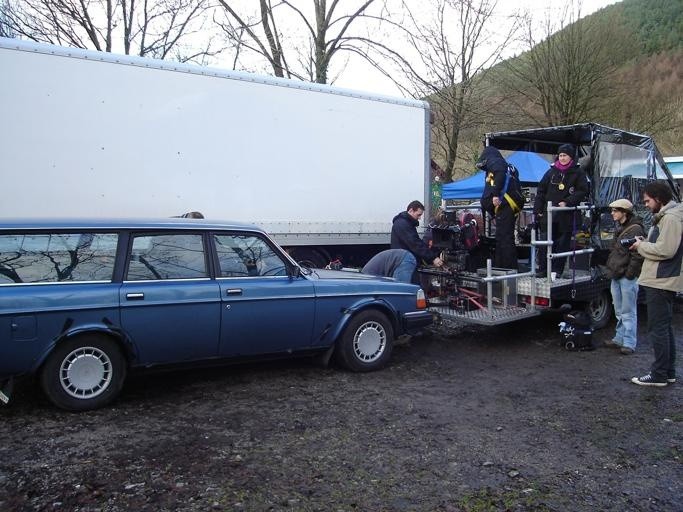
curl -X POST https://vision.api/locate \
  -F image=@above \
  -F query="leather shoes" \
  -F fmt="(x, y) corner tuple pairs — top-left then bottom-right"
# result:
(555, 273), (561, 279)
(530, 271), (547, 279)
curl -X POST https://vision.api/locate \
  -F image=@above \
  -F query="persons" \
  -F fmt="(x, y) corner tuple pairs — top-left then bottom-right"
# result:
(475, 147), (525, 274)
(604, 199), (646, 355)
(361, 249), (417, 346)
(391, 201), (444, 286)
(533, 144), (588, 278)
(422, 210), (446, 249)
(630, 183), (682, 386)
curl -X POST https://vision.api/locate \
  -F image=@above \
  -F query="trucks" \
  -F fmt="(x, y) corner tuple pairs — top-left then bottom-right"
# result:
(430, 123), (683, 330)
(2, 40), (429, 272)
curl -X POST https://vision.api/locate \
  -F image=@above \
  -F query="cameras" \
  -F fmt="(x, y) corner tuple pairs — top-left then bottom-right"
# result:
(426, 211), (478, 270)
(620, 237), (642, 248)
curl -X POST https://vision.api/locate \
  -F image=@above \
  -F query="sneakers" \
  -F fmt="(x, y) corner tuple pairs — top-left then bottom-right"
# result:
(668, 377), (676, 383)
(621, 346), (633, 354)
(604, 339), (619, 348)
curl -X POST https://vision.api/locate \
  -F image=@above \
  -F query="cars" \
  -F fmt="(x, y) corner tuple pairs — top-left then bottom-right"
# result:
(0, 218), (434, 411)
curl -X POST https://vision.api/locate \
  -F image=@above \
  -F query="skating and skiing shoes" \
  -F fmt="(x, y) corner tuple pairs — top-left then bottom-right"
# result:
(631, 371), (668, 386)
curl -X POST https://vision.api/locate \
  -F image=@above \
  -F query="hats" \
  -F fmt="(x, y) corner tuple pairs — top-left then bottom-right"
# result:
(558, 143), (574, 158)
(609, 199), (633, 209)
